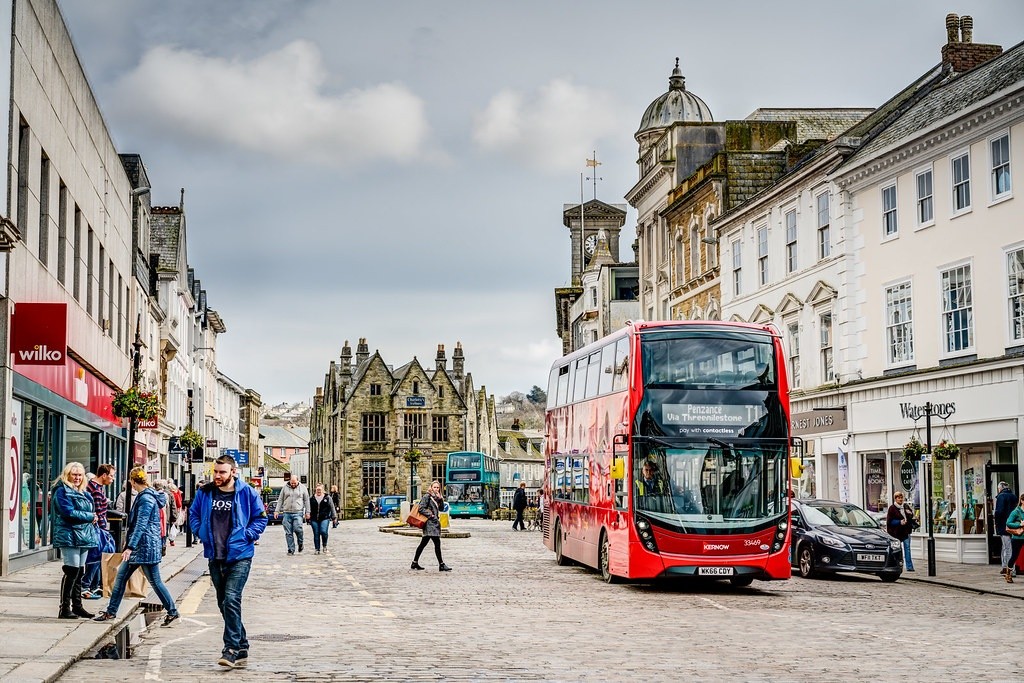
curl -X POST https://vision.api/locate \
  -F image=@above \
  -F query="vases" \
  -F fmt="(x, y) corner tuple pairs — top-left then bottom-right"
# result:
(905, 450), (921, 460)
(116, 406), (147, 418)
(935, 450), (959, 460)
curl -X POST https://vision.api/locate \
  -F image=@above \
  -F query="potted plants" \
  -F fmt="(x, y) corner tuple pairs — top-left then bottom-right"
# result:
(405, 449), (420, 462)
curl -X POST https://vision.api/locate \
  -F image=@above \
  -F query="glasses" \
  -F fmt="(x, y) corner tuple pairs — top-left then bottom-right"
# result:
(645, 469), (653, 472)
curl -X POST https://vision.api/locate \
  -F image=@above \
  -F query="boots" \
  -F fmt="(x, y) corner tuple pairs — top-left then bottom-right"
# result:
(72, 566), (96, 618)
(58, 565), (80, 619)
(1005, 567), (1014, 583)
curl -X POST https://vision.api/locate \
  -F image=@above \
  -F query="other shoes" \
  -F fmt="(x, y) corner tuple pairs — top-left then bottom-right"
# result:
(236, 649), (248, 663)
(1000, 568), (1007, 575)
(81, 587), (101, 599)
(288, 549), (295, 555)
(218, 647), (238, 666)
(163, 545), (166, 556)
(298, 546), (304, 552)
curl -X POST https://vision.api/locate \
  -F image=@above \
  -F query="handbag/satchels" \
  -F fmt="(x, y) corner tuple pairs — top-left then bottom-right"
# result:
(1006, 523), (1024, 535)
(911, 519), (920, 529)
(101, 552), (149, 598)
(406, 504), (433, 528)
(86, 528), (116, 565)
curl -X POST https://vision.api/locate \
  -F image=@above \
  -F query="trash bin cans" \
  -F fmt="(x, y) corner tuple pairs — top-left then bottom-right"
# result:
(106, 510), (123, 554)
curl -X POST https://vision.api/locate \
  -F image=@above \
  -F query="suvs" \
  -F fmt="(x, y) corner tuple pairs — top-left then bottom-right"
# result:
(375, 495), (406, 518)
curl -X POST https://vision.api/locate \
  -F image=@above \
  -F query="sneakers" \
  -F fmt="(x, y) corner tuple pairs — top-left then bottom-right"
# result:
(323, 546), (327, 553)
(314, 549), (321, 555)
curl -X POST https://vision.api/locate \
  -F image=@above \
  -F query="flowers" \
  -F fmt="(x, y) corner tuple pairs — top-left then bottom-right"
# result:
(180, 428), (203, 448)
(902, 435), (925, 458)
(110, 386), (167, 429)
(933, 440), (961, 459)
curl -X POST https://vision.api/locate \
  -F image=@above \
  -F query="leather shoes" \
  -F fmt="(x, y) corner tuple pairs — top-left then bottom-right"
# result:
(439, 563), (452, 571)
(411, 562), (424, 570)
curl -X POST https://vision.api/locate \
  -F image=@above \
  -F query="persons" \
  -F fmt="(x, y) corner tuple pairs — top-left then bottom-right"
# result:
(274, 475), (340, 555)
(634, 461), (672, 497)
(50, 462), (205, 626)
(368, 501), (374, 519)
(887, 491), (920, 571)
(994, 481), (1024, 583)
(189, 455), (269, 667)
(512, 482), (527, 532)
(536, 489), (544, 532)
(406, 481), (453, 571)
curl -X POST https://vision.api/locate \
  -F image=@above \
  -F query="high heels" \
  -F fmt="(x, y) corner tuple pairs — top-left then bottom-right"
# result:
(161, 614), (182, 627)
(93, 611), (116, 623)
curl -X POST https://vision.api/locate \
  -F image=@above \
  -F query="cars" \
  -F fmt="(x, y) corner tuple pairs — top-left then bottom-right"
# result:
(766, 498), (904, 583)
(265, 501), (284, 526)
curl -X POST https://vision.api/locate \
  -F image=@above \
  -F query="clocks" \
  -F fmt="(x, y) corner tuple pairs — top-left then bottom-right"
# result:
(584, 234), (598, 255)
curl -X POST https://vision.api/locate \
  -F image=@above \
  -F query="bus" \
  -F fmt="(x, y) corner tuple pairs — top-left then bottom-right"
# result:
(444, 452), (501, 519)
(543, 319), (804, 586)
